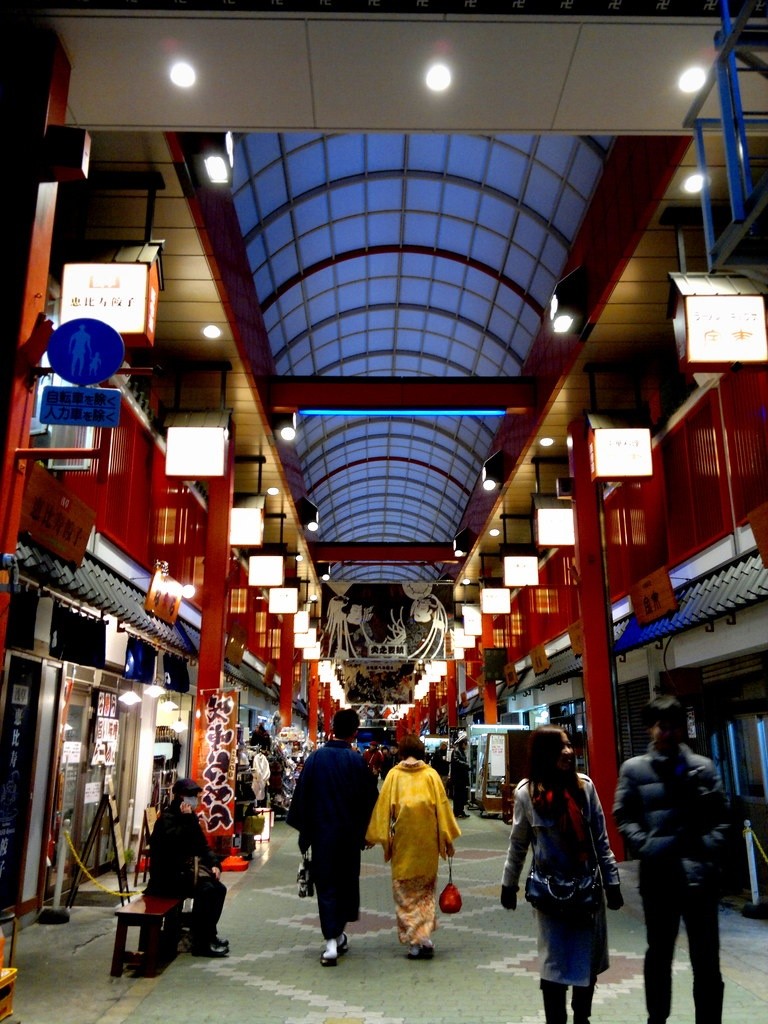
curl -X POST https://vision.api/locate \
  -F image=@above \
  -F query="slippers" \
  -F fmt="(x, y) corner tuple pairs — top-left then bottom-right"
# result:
(338, 932), (347, 951)
(408, 943), (433, 960)
(320, 950), (338, 967)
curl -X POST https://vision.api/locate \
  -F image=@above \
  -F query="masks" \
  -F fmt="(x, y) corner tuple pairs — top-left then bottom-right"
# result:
(181, 794), (200, 811)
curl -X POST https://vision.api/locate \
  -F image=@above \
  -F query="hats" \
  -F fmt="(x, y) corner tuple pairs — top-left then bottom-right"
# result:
(370, 741), (377, 746)
(440, 740), (448, 747)
(171, 780), (203, 796)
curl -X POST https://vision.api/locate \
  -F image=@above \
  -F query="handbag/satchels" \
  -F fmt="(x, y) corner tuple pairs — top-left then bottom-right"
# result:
(297, 852), (314, 898)
(439, 879), (463, 914)
(524, 865), (600, 921)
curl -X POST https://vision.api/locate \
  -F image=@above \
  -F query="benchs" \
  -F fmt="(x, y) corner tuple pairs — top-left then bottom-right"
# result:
(111, 896), (184, 977)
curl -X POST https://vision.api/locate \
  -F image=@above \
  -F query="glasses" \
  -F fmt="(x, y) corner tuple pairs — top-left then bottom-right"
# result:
(463, 742), (468, 746)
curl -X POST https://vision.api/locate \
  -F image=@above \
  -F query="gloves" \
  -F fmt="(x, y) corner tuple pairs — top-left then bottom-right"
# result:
(605, 884), (625, 912)
(501, 885), (518, 910)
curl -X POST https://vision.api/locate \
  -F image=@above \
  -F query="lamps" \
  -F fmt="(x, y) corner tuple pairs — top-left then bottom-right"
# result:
(162, 404), (654, 717)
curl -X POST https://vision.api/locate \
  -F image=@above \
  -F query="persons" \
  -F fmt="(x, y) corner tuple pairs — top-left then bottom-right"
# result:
(612, 695), (731, 1024)
(365, 733), (461, 960)
(500, 723), (624, 1024)
(285, 709), (379, 967)
(363, 741), (384, 777)
(451, 739), (472, 819)
(383, 742), (402, 781)
(379, 746), (390, 780)
(431, 742), (450, 786)
(141, 778), (229, 958)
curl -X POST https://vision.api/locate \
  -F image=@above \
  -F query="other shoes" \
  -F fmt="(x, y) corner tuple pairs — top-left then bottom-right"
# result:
(192, 943), (229, 957)
(212, 934), (230, 947)
(456, 813), (471, 820)
